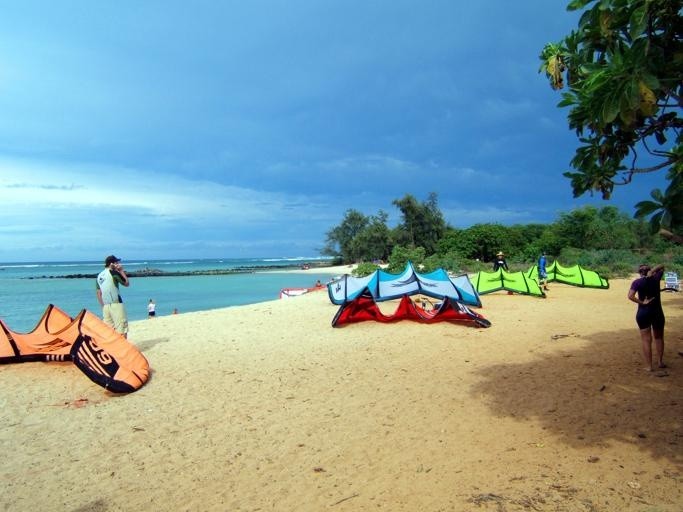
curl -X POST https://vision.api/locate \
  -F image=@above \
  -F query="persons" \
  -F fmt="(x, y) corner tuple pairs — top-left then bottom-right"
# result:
(173, 308), (178, 314)
(316, 280), (321, 286)
(147, 300), (155, 319)
(538, 251), (550, 290)
(96, 255), (129, 340)
(493, 251), (509, 272)
(628, 264), (666, 372)
(331, 278), (335, 282)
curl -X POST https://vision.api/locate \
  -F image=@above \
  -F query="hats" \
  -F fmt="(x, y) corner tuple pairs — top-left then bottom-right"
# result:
(497, 252), (503, 255)
(105, 255), (119, 266)
